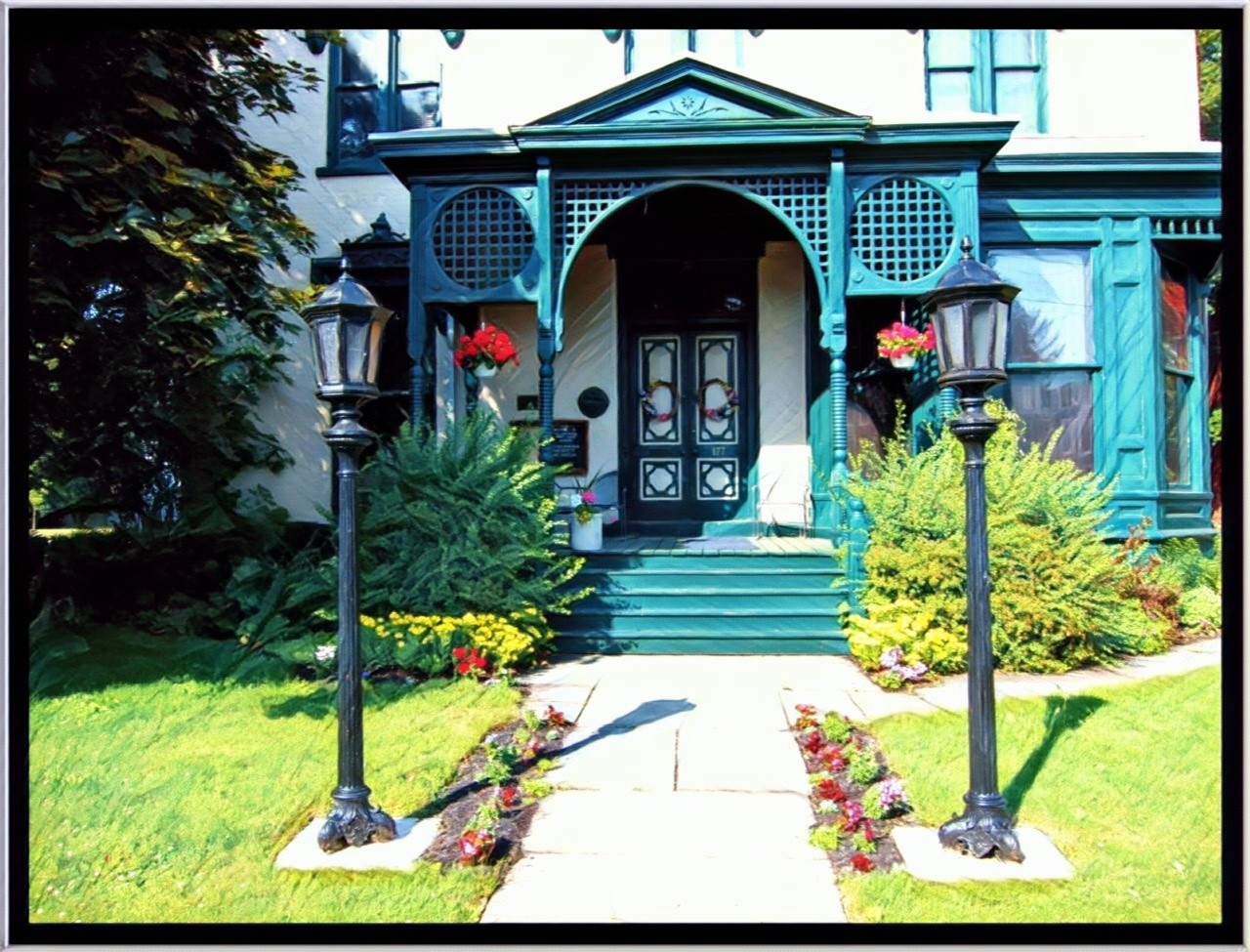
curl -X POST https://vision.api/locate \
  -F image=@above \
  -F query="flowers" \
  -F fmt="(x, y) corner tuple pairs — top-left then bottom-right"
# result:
(451, 324), (521, 371)
(571, 489), (606, 524)
(875, 322), (938, 358)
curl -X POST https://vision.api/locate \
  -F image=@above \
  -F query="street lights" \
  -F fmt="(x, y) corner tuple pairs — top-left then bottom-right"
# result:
(296, 258), (399, 855)
(921, 232), (1023, 866)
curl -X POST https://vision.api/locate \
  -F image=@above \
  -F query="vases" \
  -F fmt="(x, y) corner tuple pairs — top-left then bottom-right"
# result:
(569, 514), (605, 551)
(888, 351), (916, 370)
(472, 358), (498, 377)
